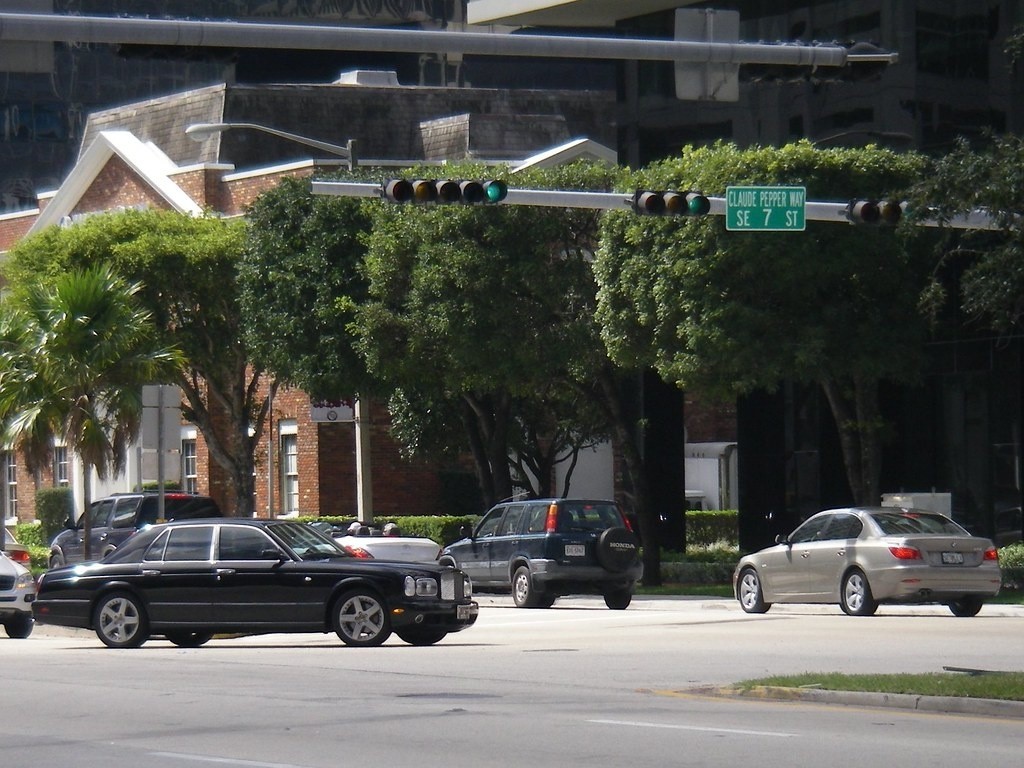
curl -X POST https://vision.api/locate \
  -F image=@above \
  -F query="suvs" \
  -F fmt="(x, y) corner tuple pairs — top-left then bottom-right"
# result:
(47, 488), (226, 574)
(436, 499), (641, 611)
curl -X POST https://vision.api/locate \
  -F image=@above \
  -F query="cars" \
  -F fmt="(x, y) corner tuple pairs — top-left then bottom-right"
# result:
(33, 517), (480, 649)
(732, 506), (1003, 617)
(0, 553), (36, 639)
(3, 528), (32, 572)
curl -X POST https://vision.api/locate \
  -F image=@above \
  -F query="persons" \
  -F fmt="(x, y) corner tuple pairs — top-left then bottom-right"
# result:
(348, 522), (398, 535)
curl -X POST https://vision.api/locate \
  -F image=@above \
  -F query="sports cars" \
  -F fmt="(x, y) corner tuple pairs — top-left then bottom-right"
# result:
(288, 519), (443, 563)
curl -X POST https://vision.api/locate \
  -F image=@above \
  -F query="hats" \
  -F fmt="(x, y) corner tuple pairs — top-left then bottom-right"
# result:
(347, 522), (361, 530)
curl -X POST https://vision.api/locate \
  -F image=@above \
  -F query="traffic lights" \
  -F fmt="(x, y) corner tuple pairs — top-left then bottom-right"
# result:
(376, 173), (511, 211)
(620, 188), (712, 220)
(843, 199), (906, 227)
